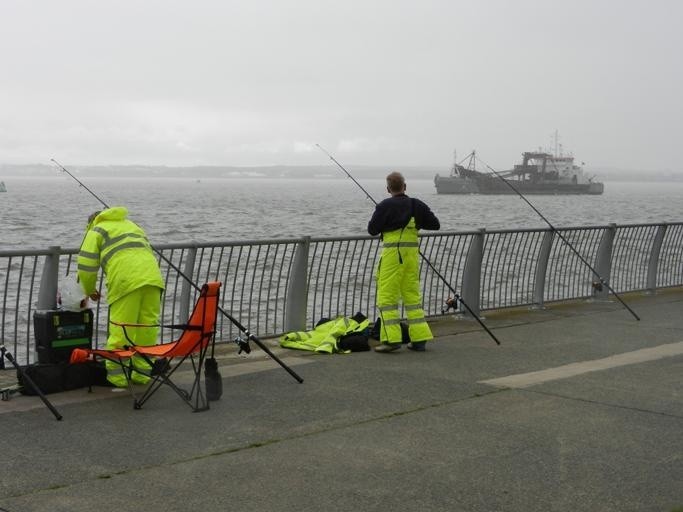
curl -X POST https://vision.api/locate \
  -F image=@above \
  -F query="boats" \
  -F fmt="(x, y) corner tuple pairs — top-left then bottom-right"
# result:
(434, 127), (604, 196)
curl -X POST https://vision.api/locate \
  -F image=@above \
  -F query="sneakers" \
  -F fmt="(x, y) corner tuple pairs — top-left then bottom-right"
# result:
(407, 339), (427, 351)
(374, 343), (402, 354)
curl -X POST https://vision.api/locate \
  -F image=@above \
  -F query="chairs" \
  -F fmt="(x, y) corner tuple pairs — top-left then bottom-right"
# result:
(84, 281), (223, 412)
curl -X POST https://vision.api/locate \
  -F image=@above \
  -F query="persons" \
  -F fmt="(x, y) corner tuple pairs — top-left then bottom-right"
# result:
(75, 211), (162, 394)
(366, 173), (441, 353)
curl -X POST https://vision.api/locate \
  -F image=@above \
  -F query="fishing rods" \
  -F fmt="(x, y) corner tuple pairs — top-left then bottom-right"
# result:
(488, 165), (641, 322)
(0, 342), (62, 421)
(315, 143), (501, 346)
(50, 158), (304, 384)
